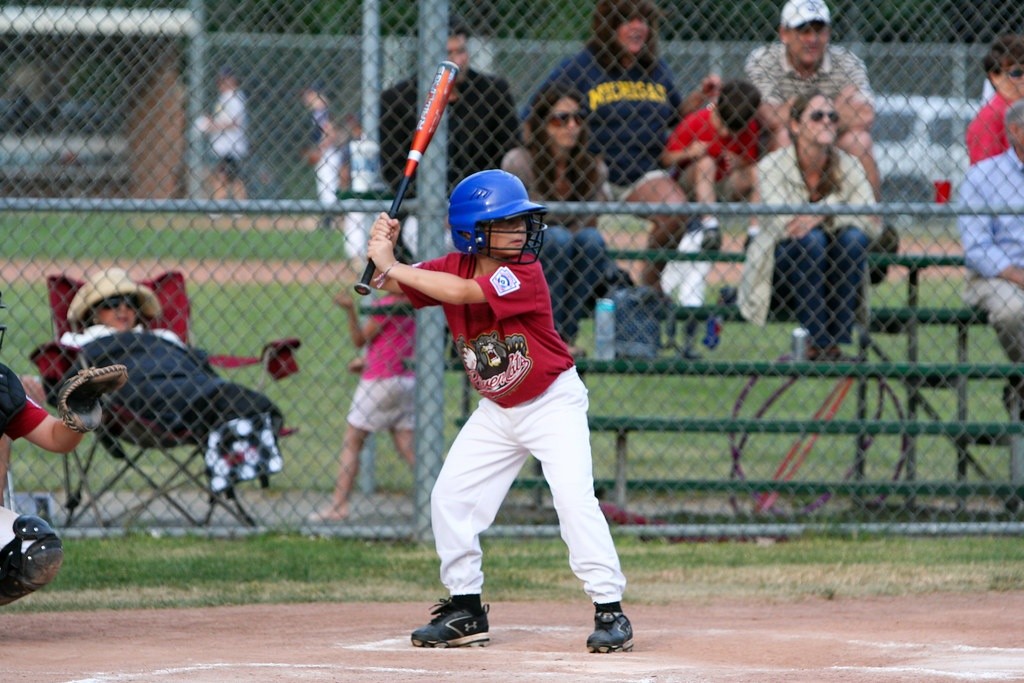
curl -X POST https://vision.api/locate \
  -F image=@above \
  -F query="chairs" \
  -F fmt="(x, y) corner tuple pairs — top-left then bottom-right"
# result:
(26, 269), (300, 528)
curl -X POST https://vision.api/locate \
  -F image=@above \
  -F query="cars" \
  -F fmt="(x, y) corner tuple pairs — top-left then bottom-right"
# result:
(865, 92), (987, 220)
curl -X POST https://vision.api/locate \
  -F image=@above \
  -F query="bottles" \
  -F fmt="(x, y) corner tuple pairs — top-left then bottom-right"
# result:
(592, 298), (616, 362)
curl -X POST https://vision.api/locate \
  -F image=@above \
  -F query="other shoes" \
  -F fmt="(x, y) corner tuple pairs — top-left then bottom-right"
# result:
(307, 508), (349, 526)
(805, 340), (842, 362)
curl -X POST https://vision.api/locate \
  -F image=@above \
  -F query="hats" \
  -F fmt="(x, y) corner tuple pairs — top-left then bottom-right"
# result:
(66, 266), (160, 325)
(781, 0), (831, 31)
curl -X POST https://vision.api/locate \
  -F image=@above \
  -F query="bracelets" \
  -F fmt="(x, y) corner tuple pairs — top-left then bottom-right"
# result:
(374, 261), (399, 289)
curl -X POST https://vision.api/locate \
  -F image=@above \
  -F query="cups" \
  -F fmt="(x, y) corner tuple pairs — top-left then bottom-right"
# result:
(933, 179), (951, 202)
(351, 142), (380, 191)
(793, 326), (810, 362)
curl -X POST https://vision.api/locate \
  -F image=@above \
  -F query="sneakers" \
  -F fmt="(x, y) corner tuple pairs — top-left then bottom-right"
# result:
(586, 608), (632, 654)
(410, 596), (490, 650)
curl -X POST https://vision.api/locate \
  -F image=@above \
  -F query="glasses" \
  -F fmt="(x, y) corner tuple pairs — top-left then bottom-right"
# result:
(996, 67), (1024, 76)
(541, 111), (586, 128)
(99, 296), (138, 311)
(794, 110), (838, 125)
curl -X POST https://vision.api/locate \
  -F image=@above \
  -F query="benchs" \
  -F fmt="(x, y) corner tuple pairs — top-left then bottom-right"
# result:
(358, 249), (1024, 521)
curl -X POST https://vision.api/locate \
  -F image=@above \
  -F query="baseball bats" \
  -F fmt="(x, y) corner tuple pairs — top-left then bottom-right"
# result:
(352, 59), (460, 296)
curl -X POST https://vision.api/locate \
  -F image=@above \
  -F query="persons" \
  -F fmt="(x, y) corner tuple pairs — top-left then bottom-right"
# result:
(958, 100), (1024, 413)
(501, 84), (609, 358)
(735, 89), (879, 362)
(61, 266), (283, 431)
(308, 241), (416, 521)
(366, 169), (634, 653)
(207, 68), (247, 218)
(298, 84), (371, 273)
(740, 0), (901, 286)
(379, 14), (522, 260)
(662, 76), (764, 253)
(519, 0), (721, 297)
(967, 33), (1024, 163)
(0, 291), (128, 607)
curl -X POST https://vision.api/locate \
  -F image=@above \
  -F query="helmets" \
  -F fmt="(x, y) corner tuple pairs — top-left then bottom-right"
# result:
(447, 169), (546, 255)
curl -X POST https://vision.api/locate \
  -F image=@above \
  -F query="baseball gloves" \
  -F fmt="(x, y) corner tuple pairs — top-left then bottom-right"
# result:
(57, 364), (131, 433)
